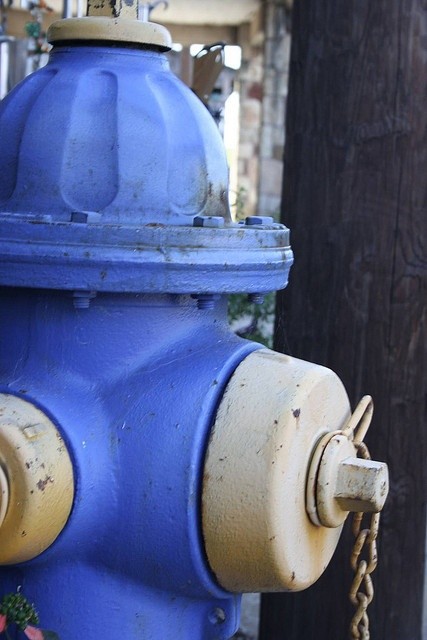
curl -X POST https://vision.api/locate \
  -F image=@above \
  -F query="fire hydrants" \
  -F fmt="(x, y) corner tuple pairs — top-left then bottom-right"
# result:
(0, 0), (389, 635)
